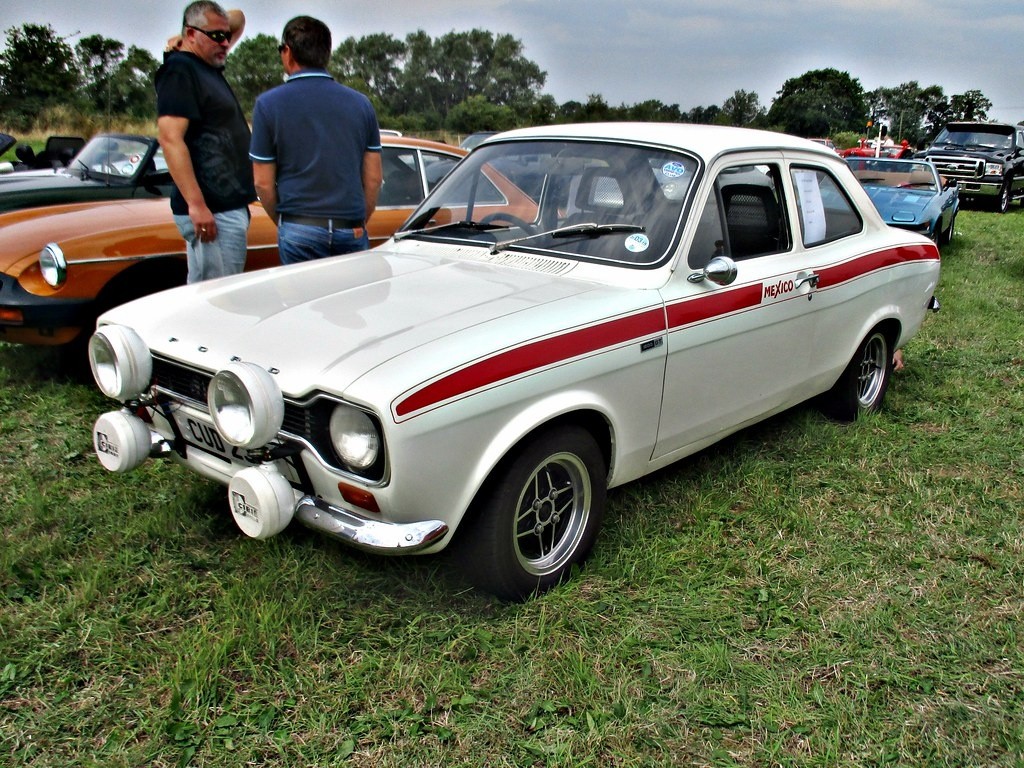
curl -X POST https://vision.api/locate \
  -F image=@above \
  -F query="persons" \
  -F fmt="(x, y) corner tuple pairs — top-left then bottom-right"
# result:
(870, 137), (879, 149)
(250, 15), (382, 267)
(154, 0), (259, 284)
(883, 135), (895, 152)
(892, 349), (904, 371)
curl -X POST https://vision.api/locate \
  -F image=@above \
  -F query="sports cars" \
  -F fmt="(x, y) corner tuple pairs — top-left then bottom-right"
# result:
(797, 158), (960, 252)
(0, 148), (562, 349)
(7, 132), (179, 217)
(0, 133), (85, 174)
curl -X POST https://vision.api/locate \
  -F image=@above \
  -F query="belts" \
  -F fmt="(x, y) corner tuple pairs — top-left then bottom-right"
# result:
(281, 213), (365, 229)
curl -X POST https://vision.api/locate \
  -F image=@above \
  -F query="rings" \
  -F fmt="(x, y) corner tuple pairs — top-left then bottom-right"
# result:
(201, 227), (206, 231)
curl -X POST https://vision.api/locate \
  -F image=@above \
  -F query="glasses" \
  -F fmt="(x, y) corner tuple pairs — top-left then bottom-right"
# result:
(184, 24), (232, 43)
(278, 43), (286, 54)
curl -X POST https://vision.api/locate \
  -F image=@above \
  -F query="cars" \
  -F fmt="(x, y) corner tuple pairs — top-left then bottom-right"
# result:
(89, 121), (939, 601)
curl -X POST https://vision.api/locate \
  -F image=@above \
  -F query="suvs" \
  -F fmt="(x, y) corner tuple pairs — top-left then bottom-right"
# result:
(913, 121), (1024, 213)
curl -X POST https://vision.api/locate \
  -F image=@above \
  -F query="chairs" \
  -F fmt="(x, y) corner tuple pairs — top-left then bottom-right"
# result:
(543, 166), (637, 266)
(9, 144), (36, 172)
(698, 183), (786, 263)
(908, 170), (933, 192)
